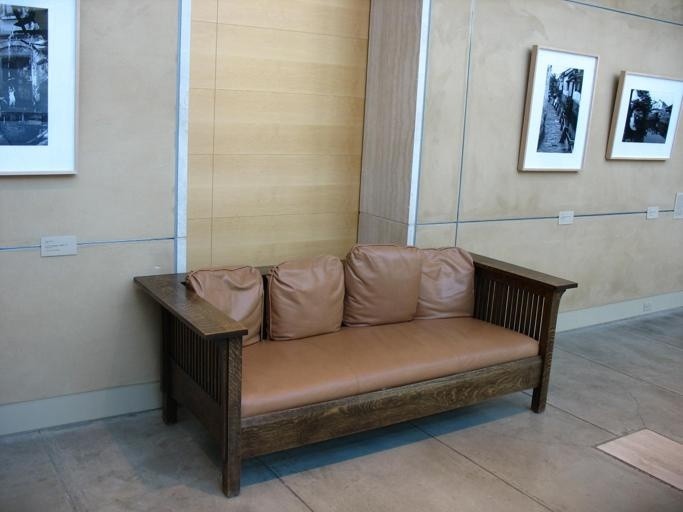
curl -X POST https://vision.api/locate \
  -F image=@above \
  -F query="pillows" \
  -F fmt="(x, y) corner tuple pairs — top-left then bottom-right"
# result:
(415, 247), (474, 319)
(343, 243), (421, 324)
(184, 264), (263, 347)
(267, 254), (345, 340)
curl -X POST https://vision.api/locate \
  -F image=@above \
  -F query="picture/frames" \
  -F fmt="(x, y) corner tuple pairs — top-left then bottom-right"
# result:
(517, 46), (597, 175)
(130, 246), (579, 498)
(606, 72), (682, 163)
(0, 0), (78, 176)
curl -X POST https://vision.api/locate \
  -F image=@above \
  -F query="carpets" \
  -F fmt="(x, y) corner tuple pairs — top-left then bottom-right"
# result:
(594, 427), (683, 491)
